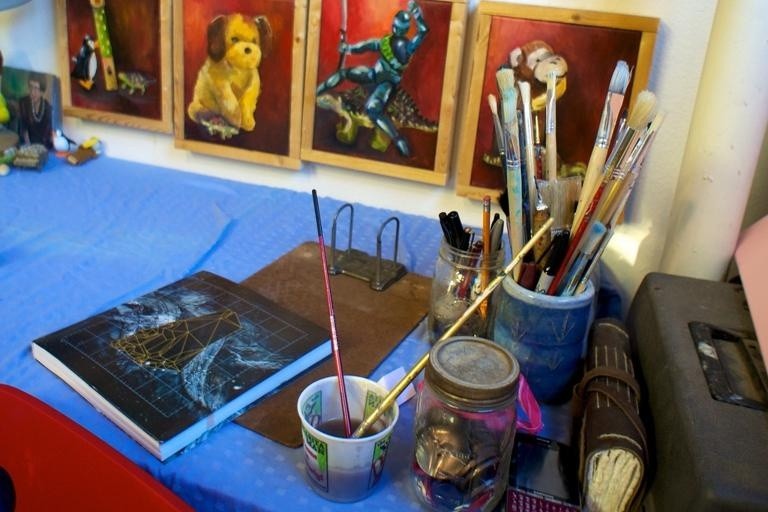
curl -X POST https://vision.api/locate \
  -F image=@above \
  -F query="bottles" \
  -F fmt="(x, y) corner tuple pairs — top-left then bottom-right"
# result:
(410, 335), (524, 512)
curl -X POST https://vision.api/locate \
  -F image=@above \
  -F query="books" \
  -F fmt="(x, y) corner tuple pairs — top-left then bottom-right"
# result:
(571, 318), (653, 511)
(29, 269), (335, 468)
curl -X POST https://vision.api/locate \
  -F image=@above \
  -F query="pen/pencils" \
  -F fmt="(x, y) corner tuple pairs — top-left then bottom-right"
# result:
(439, 195), (504, 321)
(517, 221), (614, 296)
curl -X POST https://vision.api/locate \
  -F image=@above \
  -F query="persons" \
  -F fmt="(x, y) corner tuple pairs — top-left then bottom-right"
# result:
(14, 70), (54, 152)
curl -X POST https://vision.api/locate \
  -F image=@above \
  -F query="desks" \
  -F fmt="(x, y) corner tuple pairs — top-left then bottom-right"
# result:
(1, 156), (622, 510)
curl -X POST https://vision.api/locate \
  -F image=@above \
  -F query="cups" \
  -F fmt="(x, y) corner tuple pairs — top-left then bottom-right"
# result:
(489, 265), (598, 406)
(426, 231), (507, 347)
(296, 374), (401, 505)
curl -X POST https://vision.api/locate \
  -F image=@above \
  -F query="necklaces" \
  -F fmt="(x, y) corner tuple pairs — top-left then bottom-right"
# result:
(29, 94), (47, 124)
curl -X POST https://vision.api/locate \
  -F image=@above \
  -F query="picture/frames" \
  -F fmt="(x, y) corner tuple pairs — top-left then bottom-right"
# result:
(170, 0), (309, 172)
(304, 0), (468, 187)
(53, 0), (172, 135)
(455, 0), (661, 226)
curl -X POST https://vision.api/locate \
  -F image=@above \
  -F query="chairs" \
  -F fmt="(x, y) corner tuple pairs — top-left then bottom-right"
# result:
(0, 385), (196, 512)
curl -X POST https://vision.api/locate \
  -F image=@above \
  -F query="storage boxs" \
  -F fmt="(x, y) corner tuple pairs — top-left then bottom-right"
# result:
(623, 273), (768, 510)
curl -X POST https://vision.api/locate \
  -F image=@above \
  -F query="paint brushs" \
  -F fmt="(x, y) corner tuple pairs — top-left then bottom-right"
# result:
(352, 217), (554, 435)
(488, 60), (666, 295)
(312, 188), (352, 438)
